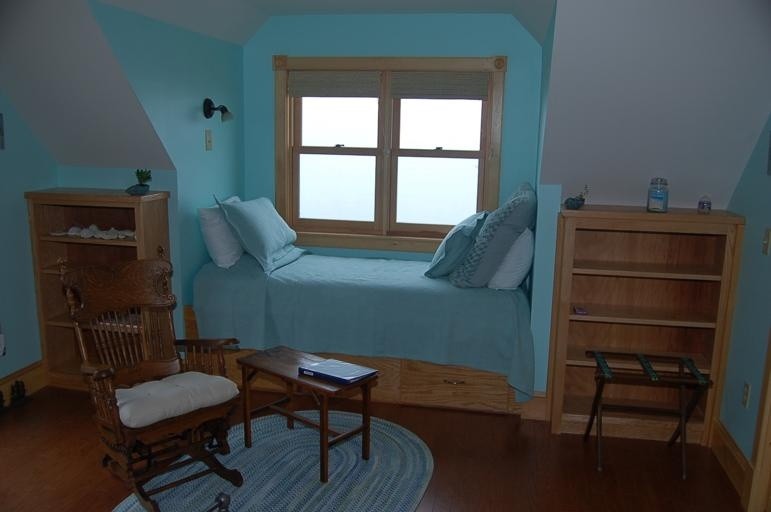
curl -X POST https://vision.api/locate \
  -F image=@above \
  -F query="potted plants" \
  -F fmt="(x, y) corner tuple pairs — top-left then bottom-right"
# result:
(124, 169), (152, 196)
(565, 185), (592, 209)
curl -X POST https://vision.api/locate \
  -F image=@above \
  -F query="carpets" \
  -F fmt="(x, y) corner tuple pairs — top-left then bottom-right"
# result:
(110, 401), (435, 511)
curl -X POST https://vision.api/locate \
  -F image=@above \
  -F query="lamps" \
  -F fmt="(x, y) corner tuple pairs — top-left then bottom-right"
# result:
(203, 98), (234, 123)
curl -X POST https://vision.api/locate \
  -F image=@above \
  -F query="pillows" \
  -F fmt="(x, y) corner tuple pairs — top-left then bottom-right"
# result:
(113, 371), (239, 429)
(196, 202), (246, 268)
(487, 225), (535, 291)
(428, 209), (489, 279)
(214, 187), (310, 276)
(451, 181), (537, 290)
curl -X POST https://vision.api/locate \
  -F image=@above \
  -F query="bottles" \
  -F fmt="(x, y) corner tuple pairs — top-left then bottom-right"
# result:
(647, 177), (670, 213)
(583, 184), (593, 209)
(699, 194), (713, 214)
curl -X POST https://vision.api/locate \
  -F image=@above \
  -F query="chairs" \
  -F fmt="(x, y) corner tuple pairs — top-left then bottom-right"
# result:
(54, 244), (244, 512)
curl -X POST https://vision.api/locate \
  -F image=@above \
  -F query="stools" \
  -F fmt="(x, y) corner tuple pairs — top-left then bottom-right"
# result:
(584, 348), (713, 482)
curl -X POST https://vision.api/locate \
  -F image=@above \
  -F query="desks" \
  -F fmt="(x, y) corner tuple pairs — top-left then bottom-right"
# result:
(234, 345), (378, 483)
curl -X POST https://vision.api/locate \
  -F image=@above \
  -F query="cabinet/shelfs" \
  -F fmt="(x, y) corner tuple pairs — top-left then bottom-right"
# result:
(185, 259), (527, 421)
(23, 185), (173, 392)
(547, 200), (746, 448)
